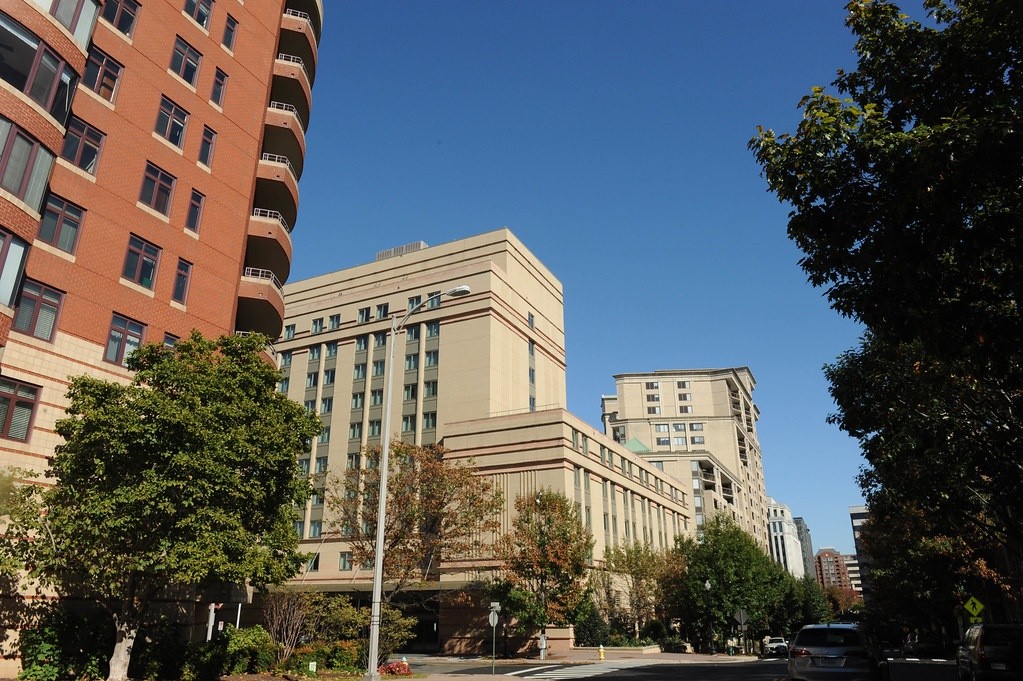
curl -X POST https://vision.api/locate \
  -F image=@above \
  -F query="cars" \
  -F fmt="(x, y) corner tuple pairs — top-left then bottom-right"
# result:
(785, 621), (879, 681)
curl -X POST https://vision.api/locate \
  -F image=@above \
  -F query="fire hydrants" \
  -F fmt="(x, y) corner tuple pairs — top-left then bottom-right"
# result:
(597, 645), (605, 662)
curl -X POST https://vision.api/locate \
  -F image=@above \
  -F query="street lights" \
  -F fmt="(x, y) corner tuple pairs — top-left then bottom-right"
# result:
(361, 285), (471, 679)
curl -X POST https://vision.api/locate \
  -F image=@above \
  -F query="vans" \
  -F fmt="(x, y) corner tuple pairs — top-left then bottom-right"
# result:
(952, 619), (1023, 681)
(766, 637), (789, 658)
